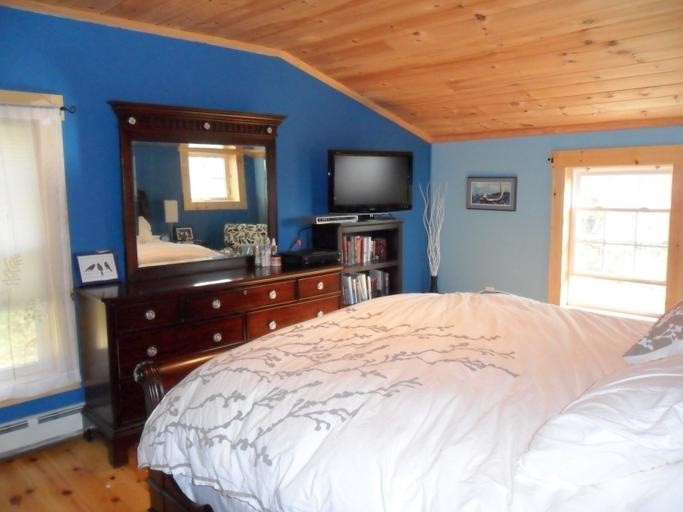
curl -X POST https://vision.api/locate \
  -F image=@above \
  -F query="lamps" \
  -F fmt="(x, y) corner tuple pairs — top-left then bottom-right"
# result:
(163, 199), (177, 243)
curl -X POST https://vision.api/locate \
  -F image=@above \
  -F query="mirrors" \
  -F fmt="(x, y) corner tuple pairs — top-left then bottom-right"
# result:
(105, 98), (287, 289)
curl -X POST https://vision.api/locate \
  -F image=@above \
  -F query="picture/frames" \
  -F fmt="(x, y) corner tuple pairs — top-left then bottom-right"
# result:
(71, 247), (122, 287)
(174, 225), (194, 241)
(467, 176), (517, 212)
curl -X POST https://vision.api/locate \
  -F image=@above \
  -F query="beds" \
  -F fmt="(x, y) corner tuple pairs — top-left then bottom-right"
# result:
(135, 191), (226, 266)
(132, 288), (680, 512)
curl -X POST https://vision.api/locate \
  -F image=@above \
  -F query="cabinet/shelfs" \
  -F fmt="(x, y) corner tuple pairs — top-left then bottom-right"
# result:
(311, 218), (403, 307)
(71, 262), (346, 468)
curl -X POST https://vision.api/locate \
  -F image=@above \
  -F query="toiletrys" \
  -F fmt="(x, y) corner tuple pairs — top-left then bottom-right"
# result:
(255, 238), (282, 267)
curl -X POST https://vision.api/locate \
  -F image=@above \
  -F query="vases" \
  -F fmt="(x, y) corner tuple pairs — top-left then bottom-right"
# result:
(430, 276), (438, 293)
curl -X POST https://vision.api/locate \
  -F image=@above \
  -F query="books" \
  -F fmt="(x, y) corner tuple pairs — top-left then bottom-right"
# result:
(343, 270), (389, 304)
(343, 235), (386, 266)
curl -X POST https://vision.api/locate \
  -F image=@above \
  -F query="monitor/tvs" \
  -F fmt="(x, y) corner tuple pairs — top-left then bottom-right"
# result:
(328, 149), (413, 220)
